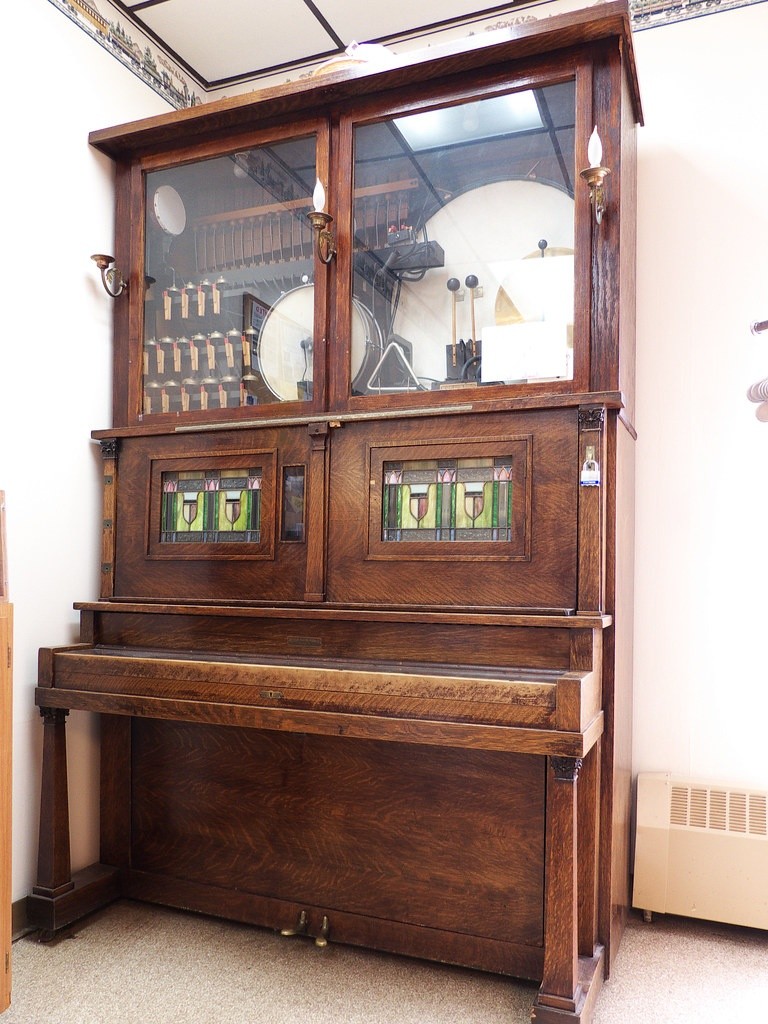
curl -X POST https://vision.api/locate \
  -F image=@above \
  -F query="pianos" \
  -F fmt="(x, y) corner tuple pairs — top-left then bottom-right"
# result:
(26, 600), (612, 1024)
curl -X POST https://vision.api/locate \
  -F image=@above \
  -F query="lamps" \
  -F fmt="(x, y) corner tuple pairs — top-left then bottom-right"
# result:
(580, 125), (610, 225)
(307, 176), (336, 264)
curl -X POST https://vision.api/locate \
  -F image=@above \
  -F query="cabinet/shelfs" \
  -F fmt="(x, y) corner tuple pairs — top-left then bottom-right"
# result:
(29, 0), (645, 1024)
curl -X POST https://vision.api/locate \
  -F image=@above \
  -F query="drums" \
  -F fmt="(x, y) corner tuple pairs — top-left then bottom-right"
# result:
(256, 282), (385, 402)
